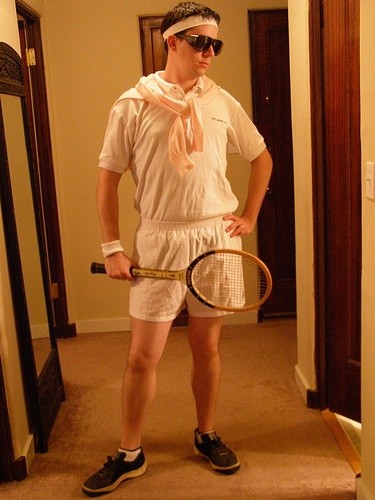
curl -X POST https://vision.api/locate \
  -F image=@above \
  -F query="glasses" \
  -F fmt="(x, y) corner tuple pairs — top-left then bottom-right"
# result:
(175, 35), (223, 56)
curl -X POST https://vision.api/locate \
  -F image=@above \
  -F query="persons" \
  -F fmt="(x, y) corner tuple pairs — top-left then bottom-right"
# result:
(81, 1), (273, 497)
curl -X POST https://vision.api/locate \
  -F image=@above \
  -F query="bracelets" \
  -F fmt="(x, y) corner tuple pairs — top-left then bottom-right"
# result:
(101, 240), (125, 258)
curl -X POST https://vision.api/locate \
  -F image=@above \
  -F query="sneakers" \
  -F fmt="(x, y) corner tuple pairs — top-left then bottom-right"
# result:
(81, 451), (147, 495)
(193, 428), (240, 472)
